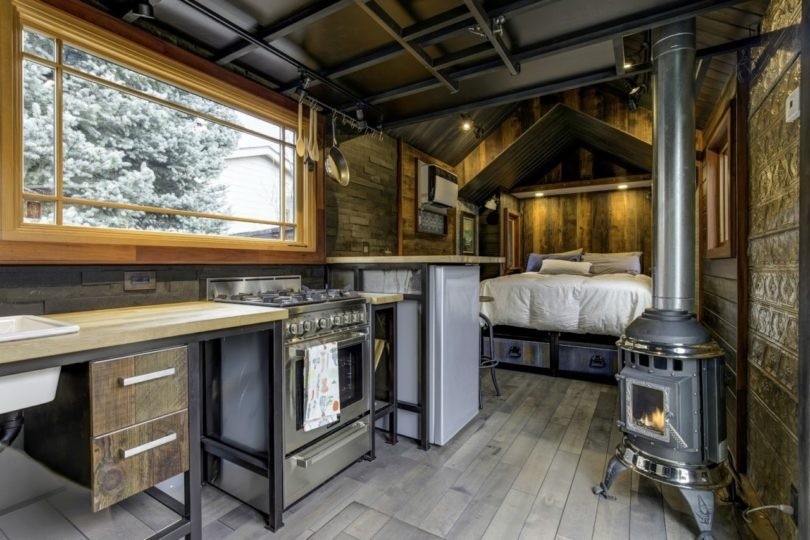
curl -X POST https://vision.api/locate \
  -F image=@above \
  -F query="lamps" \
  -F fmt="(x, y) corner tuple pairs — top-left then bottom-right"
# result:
(629, 84), (648, 111)
(460, 113), (485, 139)
(485, 194), (497, 210)
(623, 54), (641, 94)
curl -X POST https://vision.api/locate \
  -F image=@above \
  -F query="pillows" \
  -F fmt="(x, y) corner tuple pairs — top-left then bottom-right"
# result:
(527, 248), (583, 272)
(585, 251), (643, 276)
(539, 258), (593, 276)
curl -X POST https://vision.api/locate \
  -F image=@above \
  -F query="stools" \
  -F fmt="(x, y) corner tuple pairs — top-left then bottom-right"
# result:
(479, 295), (500, 410)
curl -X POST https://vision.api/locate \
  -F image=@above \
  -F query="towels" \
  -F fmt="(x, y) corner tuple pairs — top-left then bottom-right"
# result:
(302, 341), (341, 433)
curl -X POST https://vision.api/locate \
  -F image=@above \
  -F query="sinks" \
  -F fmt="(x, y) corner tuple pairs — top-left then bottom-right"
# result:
(1, 312), (80, 416)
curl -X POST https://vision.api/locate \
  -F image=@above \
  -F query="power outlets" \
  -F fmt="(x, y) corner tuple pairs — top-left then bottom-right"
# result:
(790, 485), (799, 527)
(124, 271), (157, 291)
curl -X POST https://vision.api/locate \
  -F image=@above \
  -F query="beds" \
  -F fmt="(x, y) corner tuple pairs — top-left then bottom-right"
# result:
(481, 271), (653, 385)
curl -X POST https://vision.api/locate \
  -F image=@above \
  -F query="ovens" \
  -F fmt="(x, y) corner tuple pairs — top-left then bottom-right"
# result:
(205, 328), (373, 513)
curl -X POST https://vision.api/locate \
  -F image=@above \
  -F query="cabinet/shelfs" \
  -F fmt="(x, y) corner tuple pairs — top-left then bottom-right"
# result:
(22, 346), (191, 512)
(345, 291), (404, 461)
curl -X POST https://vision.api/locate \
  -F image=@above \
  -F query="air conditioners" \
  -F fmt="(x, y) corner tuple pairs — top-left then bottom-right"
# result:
(421, 164), (459, 209)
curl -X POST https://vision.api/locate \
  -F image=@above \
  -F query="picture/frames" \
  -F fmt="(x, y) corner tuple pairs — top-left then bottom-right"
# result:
(460, 210), (476, 254)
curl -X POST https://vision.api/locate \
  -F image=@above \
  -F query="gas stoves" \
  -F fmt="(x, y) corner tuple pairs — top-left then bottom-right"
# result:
(205, 275), (368, 340)
